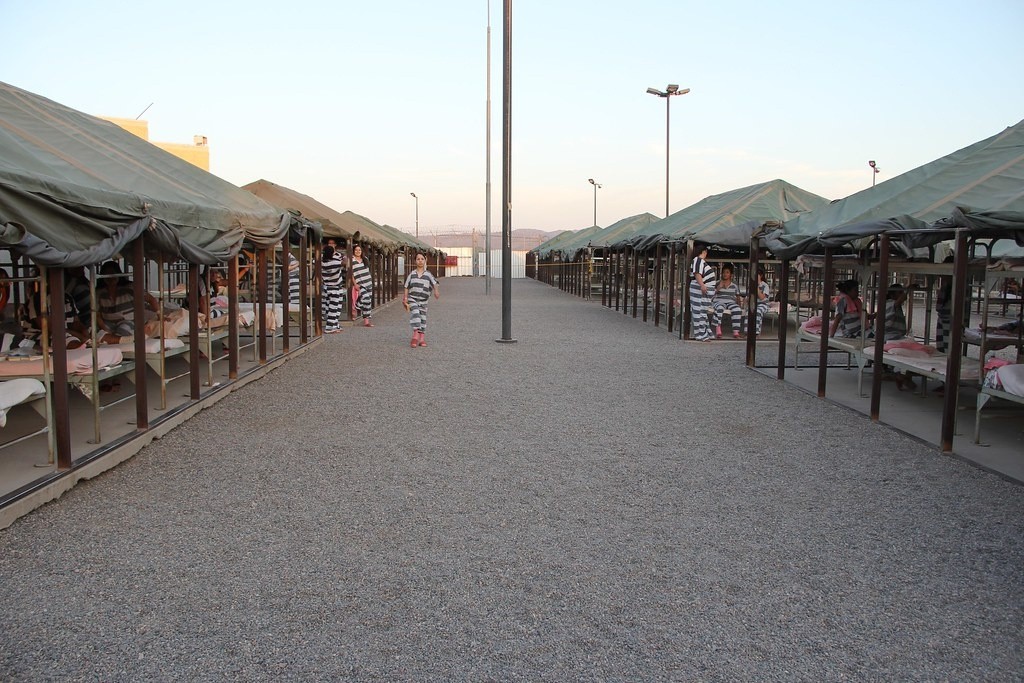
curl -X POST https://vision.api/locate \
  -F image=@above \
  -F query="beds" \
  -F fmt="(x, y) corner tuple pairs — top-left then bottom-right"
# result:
(0, 285), (317, 463)
(584, 283), (1024, 448)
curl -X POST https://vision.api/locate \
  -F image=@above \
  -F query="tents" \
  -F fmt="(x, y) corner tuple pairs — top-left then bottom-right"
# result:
(1, 83), (292, 507)
(523, 119), (1024, 455)
(237, 178), (448, 324)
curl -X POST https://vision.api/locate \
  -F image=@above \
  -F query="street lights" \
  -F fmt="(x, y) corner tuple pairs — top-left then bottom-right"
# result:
(410, 192), (418, 238)
(646, 84), (690, 217)
(588, 178), (602, 227)
(868, 160), (880, 187)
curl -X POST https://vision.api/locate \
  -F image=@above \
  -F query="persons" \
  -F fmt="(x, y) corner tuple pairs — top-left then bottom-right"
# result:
(828, 279), (874, 339)
(688, 244), (716, 341)
(180, 264), (229, 358)
(741, 266), (768, 337)
(227, 244), (305, 303)
(883, 281), (919, 390)
(319, 236), (347, 323)
(317, 245), (345, 334)
(350, 244), (374, 326)
(711, 262), (742, 338)
(979, 312), (1023, 338)
(0, 258), (162, 360)
(400, 251), (439, 347)
(933, 255), (955, 392)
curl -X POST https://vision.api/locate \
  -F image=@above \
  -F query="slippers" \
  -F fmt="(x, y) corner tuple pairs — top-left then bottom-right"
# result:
(362, 322), (375, 327)
(410, 335), (418, 348)
(323, 327), (344, 334)
(419, 340), (427, 347)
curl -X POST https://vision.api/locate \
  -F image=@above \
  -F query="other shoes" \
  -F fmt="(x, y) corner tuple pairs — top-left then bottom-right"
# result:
(733, 334), (740, 338)
(716, 333), (723, 338)
